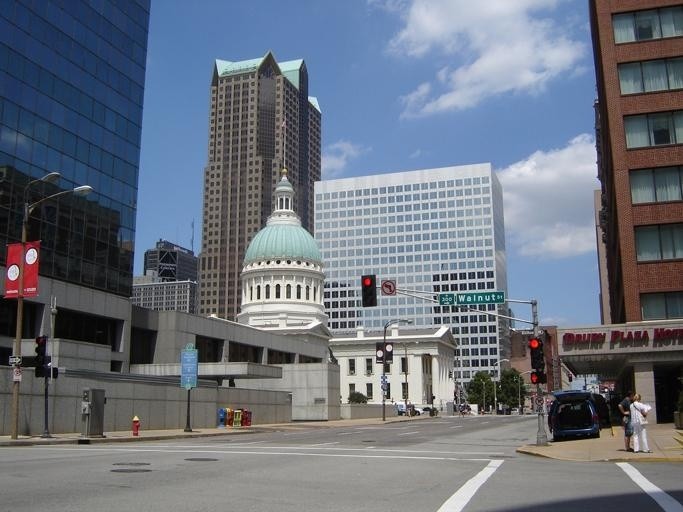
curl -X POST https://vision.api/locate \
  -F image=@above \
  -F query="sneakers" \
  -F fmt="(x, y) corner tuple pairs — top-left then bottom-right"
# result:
(626, 448), (653, 453)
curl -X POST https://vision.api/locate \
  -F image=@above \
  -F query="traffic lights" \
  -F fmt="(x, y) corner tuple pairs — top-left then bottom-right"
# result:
(568, 374), (572, 382)
(528, 338), (547, 385)
(361, 275), (377, 307)
(34, 336), (58, 378)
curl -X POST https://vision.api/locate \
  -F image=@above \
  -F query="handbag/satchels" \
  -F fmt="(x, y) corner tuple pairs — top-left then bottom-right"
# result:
(641, 410), (647, 416)
(621, 416), (628, 425)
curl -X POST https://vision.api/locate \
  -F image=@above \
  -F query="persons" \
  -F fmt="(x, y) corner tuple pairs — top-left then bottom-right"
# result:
(617, 391), (634, 452)
(458, 405), (465, 418)
(629, 393), (654, 453)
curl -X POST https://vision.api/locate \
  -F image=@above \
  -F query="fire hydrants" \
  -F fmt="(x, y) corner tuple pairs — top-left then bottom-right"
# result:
(132, 415), (140, 436)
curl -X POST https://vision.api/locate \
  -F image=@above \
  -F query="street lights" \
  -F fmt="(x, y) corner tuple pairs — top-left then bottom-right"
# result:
(11, 172), (93, 440)
(519, 371), (531, 407)
(480, 358), (510, 415)
(382, 318), (413, 421)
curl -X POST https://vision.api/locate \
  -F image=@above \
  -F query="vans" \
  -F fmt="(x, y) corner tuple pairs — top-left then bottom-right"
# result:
(548, 390), (610, 441)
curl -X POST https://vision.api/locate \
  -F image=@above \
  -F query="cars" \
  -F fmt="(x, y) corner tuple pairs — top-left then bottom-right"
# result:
(496, 405), (532, 415)
(396, 403), (431, 416)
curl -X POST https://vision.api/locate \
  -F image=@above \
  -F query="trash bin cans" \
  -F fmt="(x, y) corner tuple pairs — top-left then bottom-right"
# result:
(675, 412), (683, 429)
